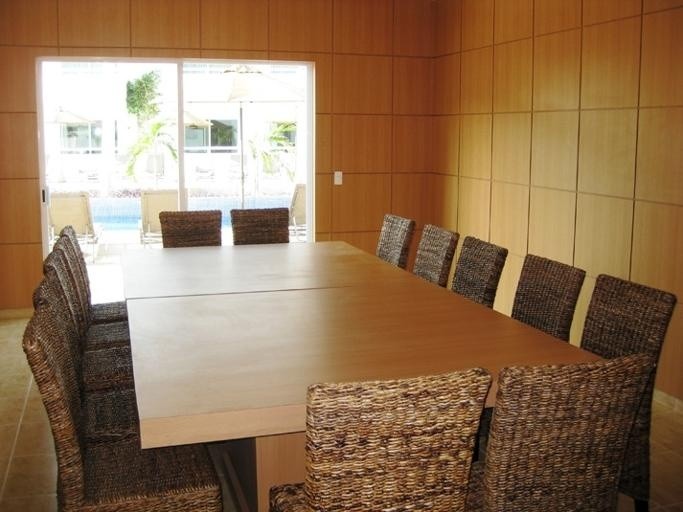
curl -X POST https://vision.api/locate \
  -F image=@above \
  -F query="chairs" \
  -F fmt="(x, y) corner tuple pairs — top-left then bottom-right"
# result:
(21, 221), (225, 510)
(228, 204), (294, 245)
(157, 209), (225, 247)
(266, 363), (496, 511)
(478, 350), (658, 510)
(373, 210), (676, 368)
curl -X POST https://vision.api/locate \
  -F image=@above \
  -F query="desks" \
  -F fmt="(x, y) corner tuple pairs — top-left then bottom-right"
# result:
(112, 240), (652, 508)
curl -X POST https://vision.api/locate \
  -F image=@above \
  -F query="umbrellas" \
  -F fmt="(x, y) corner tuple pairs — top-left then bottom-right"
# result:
(185, 64), (307, 215)
